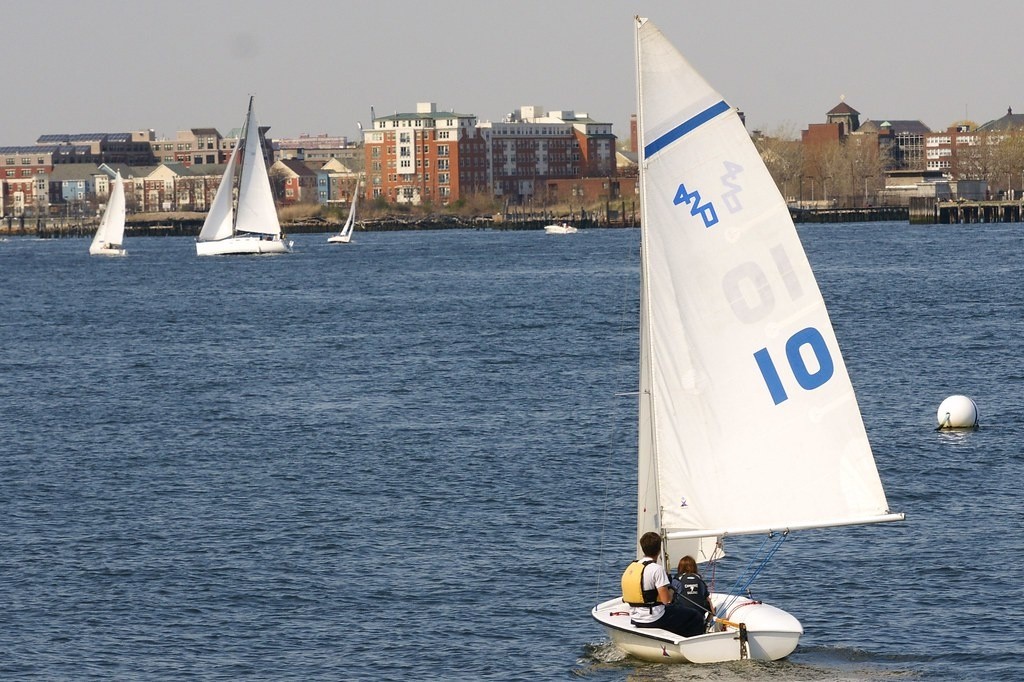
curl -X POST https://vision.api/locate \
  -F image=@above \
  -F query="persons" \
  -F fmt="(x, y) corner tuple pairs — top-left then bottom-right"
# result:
(266, 231), (286, 241)
(621, 531), (716, 638)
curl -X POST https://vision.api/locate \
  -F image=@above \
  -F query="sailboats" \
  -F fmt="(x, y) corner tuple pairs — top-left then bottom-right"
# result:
(194, 95), (295, 257)
(587, 15), (906, 668)
(88, 168), (127, 258)
(327, 174), (359, 244)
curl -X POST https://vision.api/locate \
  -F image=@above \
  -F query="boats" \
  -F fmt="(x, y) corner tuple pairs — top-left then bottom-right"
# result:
(543, 222), (577, 235)
